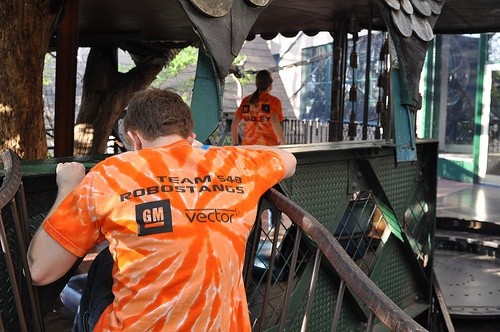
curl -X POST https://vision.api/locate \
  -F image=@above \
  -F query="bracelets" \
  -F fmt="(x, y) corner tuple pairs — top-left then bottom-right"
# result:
(233, 144), (239, 146)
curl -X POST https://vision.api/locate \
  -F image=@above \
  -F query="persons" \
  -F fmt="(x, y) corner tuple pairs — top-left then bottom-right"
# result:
(22, 87), (297, 332)
(230, 69), (287, 237)
